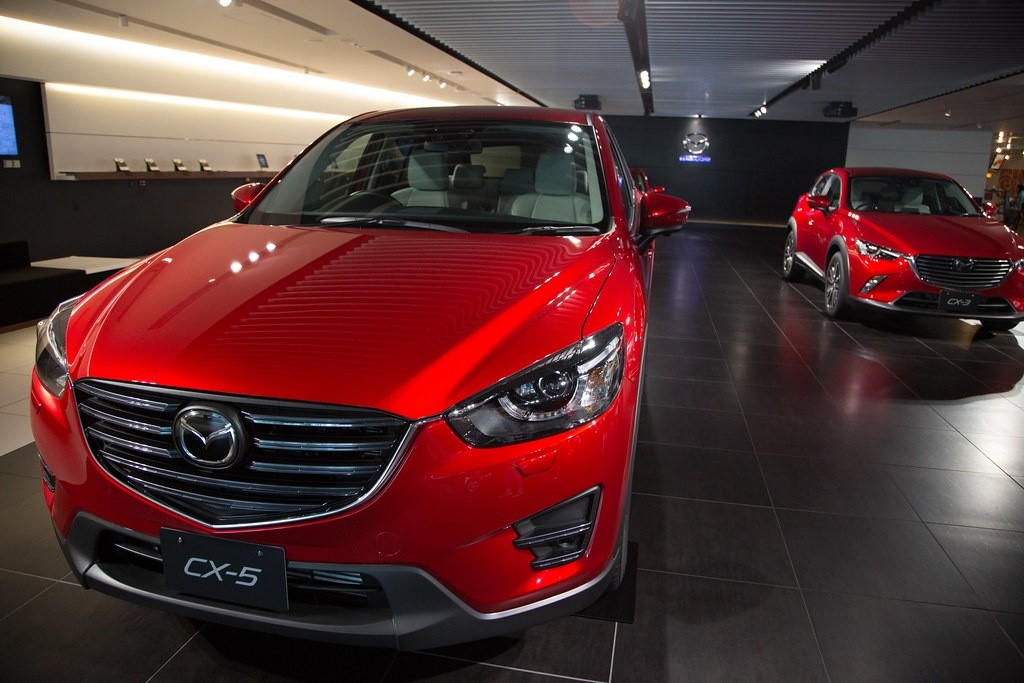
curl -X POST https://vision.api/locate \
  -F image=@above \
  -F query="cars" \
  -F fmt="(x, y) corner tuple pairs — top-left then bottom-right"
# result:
(628, 166), (666, 265)
(27, 104), (694, 652)
(782, 166), (1024, 330)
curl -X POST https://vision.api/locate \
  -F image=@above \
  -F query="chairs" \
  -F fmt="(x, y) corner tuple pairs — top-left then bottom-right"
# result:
(851, 181), (874, 211)
(448, 163), (491, 213)
(894, 188), (930, 213)
(495, 167), (537, 217)
(390, 152), (459, 210)
(509, 152), (594, 225)
(878, 187), (899, 211)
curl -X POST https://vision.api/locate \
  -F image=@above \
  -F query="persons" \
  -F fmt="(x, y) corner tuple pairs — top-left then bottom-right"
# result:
(1015, 184), (1024, 213)
